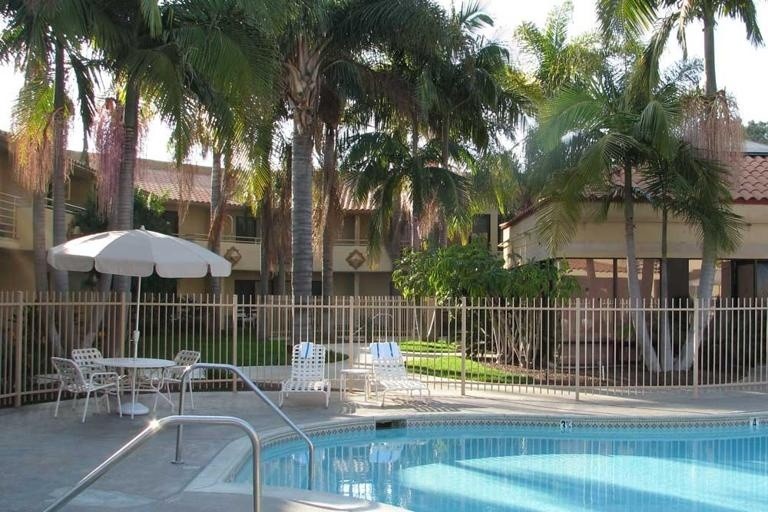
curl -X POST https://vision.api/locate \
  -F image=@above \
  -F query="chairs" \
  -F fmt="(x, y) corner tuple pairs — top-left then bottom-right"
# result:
(47, 345), (203, 423)
(367, 342), (432, 410)
(278, 339), (332, 408)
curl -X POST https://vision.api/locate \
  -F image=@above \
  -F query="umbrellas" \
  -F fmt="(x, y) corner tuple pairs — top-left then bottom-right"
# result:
(44, 223), (233, 419)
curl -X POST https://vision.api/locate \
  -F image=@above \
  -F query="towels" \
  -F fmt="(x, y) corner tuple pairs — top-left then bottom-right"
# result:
(298, 341), (314, 360)
(369, 343), (398, 360)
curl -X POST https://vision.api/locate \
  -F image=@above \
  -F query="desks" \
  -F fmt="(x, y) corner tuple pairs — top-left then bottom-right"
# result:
(339, 369), (371, 403)
